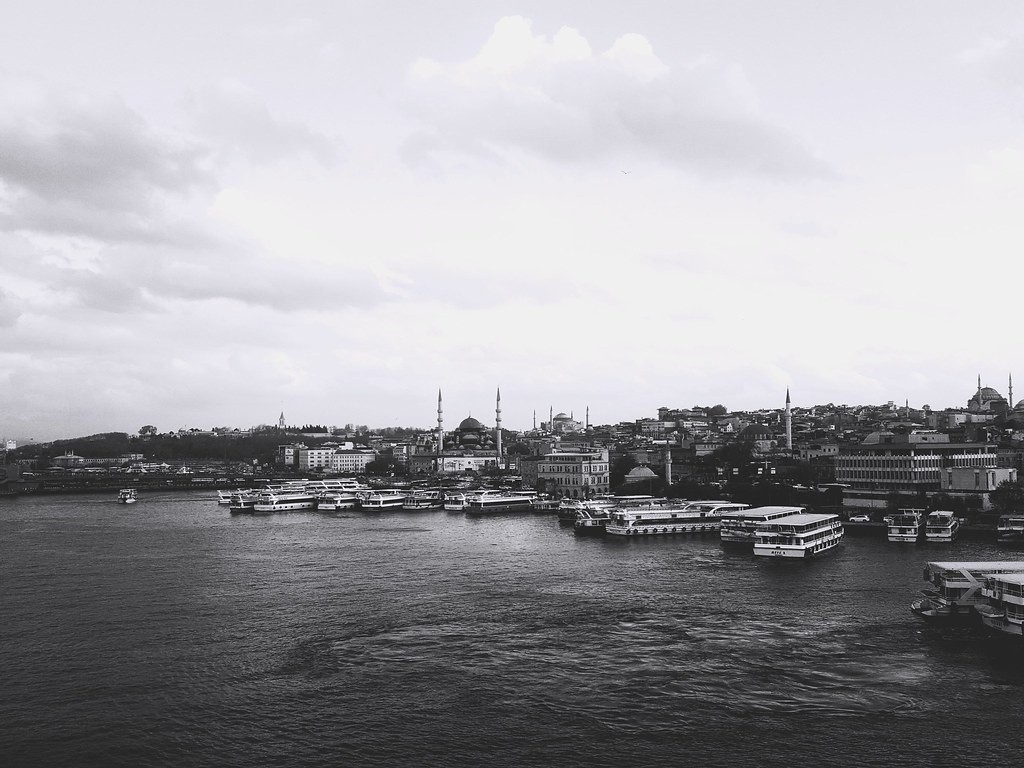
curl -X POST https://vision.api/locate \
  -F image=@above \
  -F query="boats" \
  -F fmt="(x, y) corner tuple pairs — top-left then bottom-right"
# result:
(886, 508), (926, 543)
(117, 488), (138, 504)
(924, 509), (959, 543)
(574, 517), (612, 534)
(253, 494), (317, 512)
(316, 488), (363, 511)
(975, 573), (1024, 641)
(605, 513), (722, 536)
(360, 492), (410, 511)
(997, 518), (1024, 547)
(753, 512), (846, 558)
(214, 480), (414, 511)
(464, 495), (538, 514)
(443, 493), (479, 511)
(908, 560), (1024, 617)
(996, 513), (1024, 530)
(555, 494), (752, 520)
(719, 505), (807, 542)
(402, 490), (444, 510)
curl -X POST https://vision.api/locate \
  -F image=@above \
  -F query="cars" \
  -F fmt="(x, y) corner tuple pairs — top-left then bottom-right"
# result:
(849, 515), (871, 523)
(478, 484), (535, 490)
(883, 516), (895, 522)
